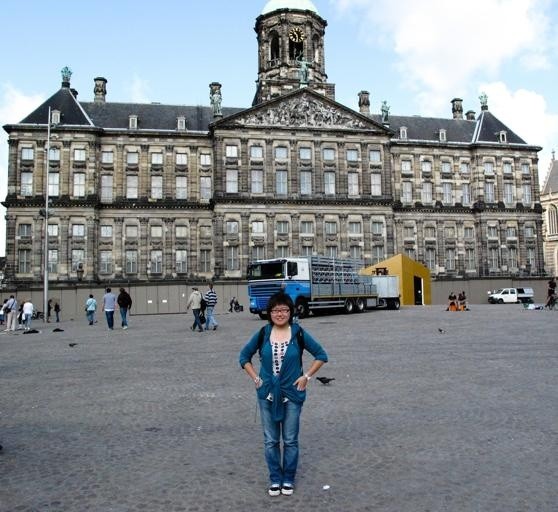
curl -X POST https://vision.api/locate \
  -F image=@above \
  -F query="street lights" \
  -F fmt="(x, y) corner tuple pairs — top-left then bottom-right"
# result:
(43, 121), (55, 320)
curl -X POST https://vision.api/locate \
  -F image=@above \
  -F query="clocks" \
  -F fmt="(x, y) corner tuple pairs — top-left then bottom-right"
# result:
(289, 27), (305, 43)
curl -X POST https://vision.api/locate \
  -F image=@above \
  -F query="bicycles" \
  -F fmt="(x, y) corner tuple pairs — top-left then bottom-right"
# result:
(547, 287), (558, 310)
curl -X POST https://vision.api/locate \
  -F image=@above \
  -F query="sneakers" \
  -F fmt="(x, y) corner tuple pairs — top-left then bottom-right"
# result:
(109, 325), (127, 329)
(4, 327), (30, 331)
(281, 483), (294, 495)
(190, 325), (217, 331)
(268, 483), (281, 497)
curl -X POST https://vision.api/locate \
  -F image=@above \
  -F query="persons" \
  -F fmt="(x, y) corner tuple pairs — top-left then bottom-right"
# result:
(239, 292), (327, 497)
(545, 278), (558, 308)
(449, 291), (466, 311)
(381, 100), (389, 124)
(296, 56), (312, 82)
(0, 288), (234, 332)
(210, 89), (222, 114)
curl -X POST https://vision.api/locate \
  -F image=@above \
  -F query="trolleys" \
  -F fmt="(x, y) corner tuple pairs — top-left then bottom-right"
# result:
(234, 303), (242, 312)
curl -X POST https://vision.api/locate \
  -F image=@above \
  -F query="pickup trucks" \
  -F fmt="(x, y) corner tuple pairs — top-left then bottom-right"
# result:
(488, 287), (534, 304)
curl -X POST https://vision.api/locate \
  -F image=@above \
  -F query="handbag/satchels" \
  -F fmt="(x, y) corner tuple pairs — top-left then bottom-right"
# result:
(85, 308), (87, 311)
(200, 299), (206, 312)
(199, 312), (206, 324)
(5, 307), (11, 314)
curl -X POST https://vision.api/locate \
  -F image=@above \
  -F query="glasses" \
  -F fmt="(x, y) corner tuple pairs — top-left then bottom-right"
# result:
(271, 309), (290, 314)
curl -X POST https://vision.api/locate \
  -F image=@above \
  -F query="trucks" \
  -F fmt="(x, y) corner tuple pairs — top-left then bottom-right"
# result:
(372, 275), (401, 310)
(246, 255), (378, 319)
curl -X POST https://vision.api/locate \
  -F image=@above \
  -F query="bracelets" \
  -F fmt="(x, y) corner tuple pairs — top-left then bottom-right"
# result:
(303, 374), (311, 382)
(255, 376), (260, 385)
